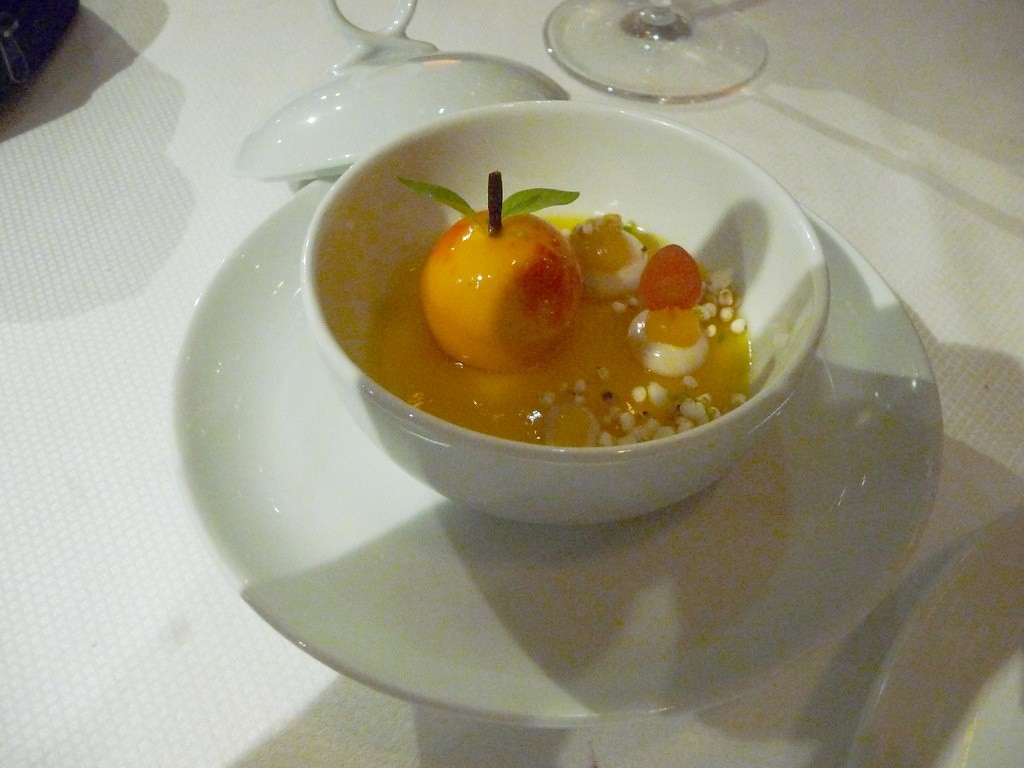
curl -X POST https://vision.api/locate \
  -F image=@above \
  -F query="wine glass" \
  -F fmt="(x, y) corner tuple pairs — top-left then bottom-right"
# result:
(545, 0), (766, 103)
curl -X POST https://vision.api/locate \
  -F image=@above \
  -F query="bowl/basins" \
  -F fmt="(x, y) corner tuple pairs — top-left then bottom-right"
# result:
(301, 100), (831, 525)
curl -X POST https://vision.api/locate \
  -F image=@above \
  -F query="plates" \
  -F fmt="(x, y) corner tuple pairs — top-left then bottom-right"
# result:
(172, 179), (944, 729)
(850, 504), (1024, 768)
